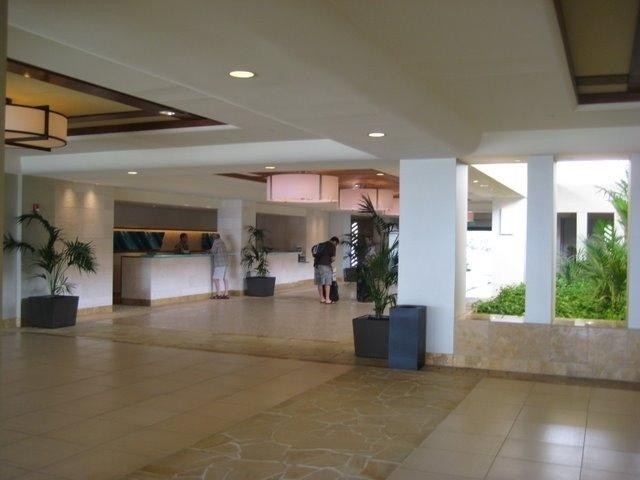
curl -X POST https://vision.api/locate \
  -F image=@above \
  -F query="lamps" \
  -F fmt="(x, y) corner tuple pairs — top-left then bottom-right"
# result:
(4, 98), (69, 152)
(266, 174), (338, 204)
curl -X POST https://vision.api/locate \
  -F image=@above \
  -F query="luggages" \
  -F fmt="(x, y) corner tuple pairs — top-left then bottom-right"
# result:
(322, 281), (339, 301)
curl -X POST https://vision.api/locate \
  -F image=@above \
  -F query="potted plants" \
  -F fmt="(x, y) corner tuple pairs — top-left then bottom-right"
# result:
(340, 194), (399, 359)
(242, 225), (276, 296)
(2, 210), (99, 329)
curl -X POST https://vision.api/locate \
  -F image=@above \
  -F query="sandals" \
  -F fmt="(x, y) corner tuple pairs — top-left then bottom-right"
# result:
(212, 294), (231, 299)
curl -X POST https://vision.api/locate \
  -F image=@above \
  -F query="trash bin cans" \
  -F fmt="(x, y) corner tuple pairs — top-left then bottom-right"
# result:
(387, 303), (427, 372)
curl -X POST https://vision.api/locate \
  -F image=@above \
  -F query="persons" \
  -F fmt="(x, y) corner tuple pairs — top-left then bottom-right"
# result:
(204, 234), (229, 299)
(173, 233), (190, 255)
(312, 236), (339, 304)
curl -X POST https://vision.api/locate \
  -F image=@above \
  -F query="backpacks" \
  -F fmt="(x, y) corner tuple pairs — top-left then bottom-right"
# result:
(311, 241), (327, 257)
